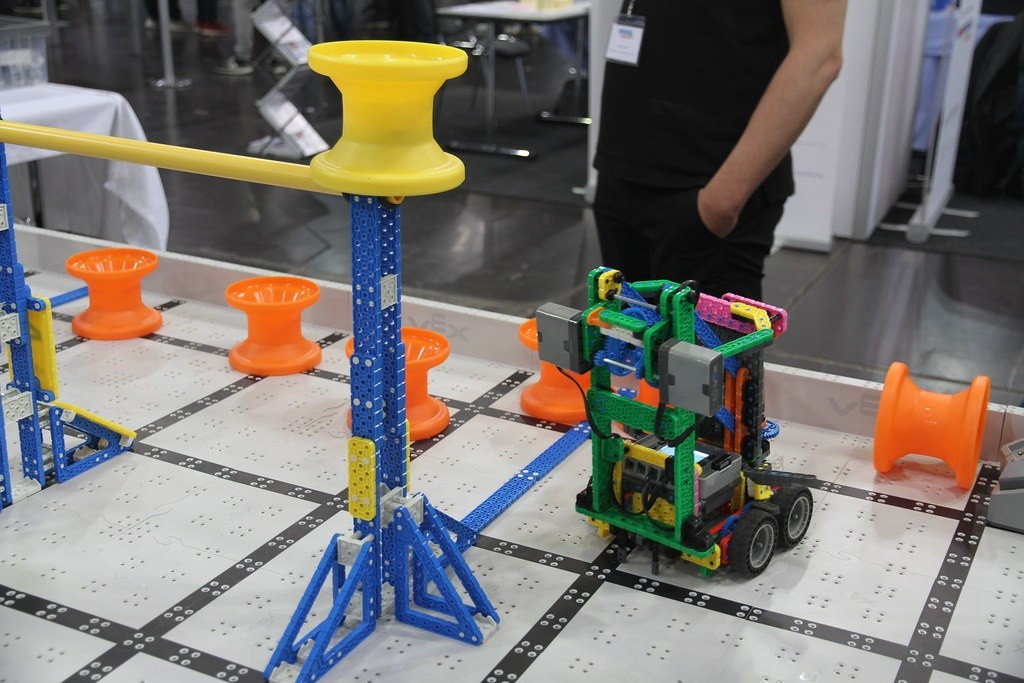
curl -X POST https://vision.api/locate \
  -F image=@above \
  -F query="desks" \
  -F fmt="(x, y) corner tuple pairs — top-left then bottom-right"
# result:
(431, 0), (593, 157)
(0, 82), (169, 251)
(0, 223), (1024, 683)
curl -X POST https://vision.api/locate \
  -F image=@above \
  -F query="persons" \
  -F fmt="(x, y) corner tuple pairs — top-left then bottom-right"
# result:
(143, 0), (441, 76)
(592, 0), (848, 301)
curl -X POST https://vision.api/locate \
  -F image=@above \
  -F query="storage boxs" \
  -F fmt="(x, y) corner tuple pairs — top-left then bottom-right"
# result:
(0, 15), (53, 93)
